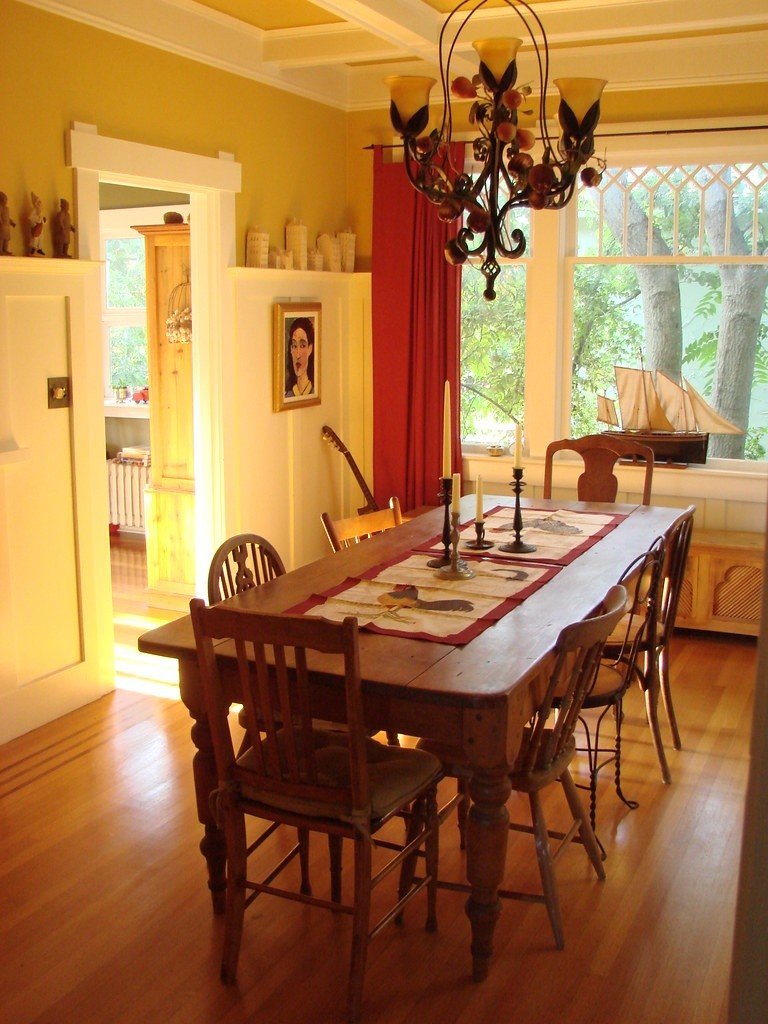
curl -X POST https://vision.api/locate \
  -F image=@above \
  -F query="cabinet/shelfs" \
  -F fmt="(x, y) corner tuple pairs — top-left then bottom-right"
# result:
(130, 223), (196, 613)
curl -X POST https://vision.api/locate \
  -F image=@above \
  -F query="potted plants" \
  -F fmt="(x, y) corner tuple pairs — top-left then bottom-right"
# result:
(112, 378), (131, 399)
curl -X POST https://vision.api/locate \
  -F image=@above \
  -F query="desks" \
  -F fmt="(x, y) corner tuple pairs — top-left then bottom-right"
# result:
(138, 494), (686, 980)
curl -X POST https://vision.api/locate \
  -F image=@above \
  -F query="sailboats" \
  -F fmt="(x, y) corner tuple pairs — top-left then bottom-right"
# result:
(594, 346), (745, 465)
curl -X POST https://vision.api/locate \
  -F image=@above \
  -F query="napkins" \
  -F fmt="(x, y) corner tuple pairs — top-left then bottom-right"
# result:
(279, 549), (563, 645)
(412, 505), (629, 566)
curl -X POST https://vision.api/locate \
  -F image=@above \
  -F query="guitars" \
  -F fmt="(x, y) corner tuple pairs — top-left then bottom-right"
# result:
(321, 424), (385, 541)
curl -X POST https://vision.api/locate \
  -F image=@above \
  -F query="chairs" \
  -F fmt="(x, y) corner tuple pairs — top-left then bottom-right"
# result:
(190, 598), (443, 1024)
(530, 535), (667, 862)
(396, 583), (627, 951)
(207, 533), (380, 760)
(321, 497), (401, 553)
(602, 504), (697, 785)
(543, 434), (654, 505)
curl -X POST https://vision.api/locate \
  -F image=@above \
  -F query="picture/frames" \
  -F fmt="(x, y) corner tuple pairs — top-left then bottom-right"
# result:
(273, 302), (322, 414)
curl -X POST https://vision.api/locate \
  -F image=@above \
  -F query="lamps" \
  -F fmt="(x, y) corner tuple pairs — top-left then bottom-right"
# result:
(362, 0), (610, 301)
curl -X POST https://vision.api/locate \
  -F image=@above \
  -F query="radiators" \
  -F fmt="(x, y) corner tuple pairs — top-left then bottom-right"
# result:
(106, 459), (151, 534)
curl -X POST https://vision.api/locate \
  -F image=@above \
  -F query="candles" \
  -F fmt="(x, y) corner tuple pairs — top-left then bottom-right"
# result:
(514, 421), (522, 469)
(451, 473), (459, 513)
(476, 474), (483, 522)
(442, 379), (451, 478)
